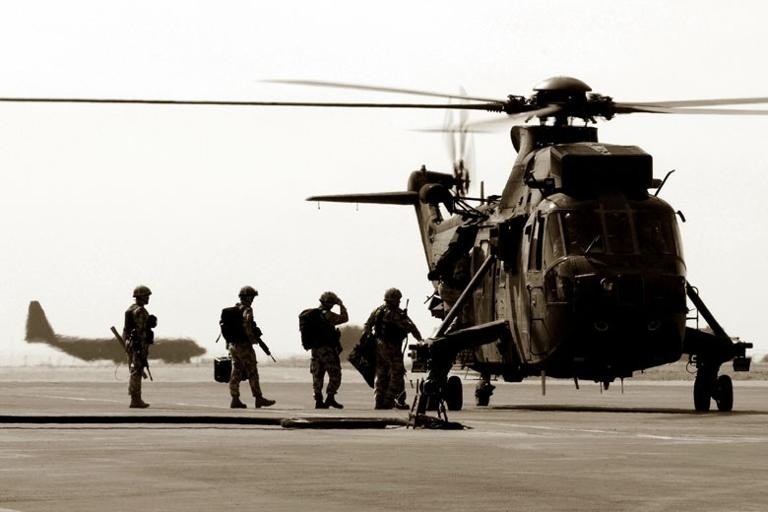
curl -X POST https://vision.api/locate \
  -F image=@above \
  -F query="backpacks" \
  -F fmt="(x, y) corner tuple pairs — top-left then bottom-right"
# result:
(219, 306), (250, 345)
(299, 307), (336, 350)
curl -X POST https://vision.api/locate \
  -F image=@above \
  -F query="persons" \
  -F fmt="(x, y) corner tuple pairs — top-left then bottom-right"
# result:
(310, 292), (348, 409)
(228, 286), (275, 408)
(438, 246), (474, 334)
(123, 286), (156, 408)
(362, 288), (424, 409)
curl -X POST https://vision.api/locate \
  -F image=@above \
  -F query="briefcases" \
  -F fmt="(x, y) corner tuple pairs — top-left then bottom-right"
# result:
(214, 357), (249, 383)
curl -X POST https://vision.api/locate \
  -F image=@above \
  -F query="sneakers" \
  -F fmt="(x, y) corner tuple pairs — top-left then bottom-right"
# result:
(129, 398), (147, 408)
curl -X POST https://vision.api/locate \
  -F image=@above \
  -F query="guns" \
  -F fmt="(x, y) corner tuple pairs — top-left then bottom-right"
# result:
(250, 321), (276, 363)
(129, 327), (152, 382)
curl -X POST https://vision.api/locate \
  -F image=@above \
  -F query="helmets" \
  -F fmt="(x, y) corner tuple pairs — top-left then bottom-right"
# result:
(133, 286), (151, 297)
(239, 285), (258, 298)
(319, 292), (339, 306)
(383, 288), (402, 302)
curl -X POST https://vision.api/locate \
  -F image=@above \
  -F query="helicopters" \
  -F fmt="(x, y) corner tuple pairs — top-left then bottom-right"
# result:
(0, 78), (768, 414)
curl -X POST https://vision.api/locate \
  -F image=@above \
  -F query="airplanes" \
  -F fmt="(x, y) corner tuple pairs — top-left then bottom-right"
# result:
(23, 301), (206, 365)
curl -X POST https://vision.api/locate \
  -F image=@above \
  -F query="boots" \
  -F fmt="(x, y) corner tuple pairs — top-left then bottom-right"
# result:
(313, 396), (327, 409)
(325, 393), (343, 410)
(253, 395), (277, 408)
(230, 395), (247, 409)
